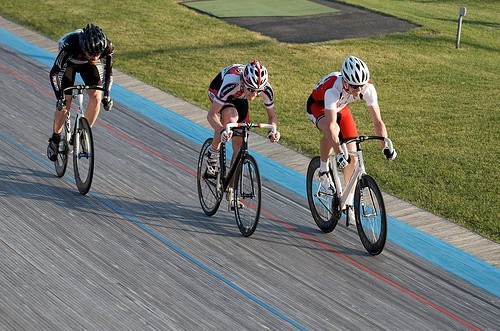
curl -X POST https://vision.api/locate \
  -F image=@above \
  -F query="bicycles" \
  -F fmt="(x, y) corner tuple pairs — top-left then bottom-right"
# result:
(51, 85), (110, 196)
(305, 135), (394, 256)
(196, 122), (276, 238)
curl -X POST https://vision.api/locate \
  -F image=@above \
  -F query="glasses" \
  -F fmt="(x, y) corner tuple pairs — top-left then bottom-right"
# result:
(247, 88), (263, 94)
(86, 52), (100, 57)
(348, 84), (366, 89)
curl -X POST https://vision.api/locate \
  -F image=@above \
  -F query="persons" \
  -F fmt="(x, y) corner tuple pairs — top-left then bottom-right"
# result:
(207, 60), (280, 210)
(307, 55), (397, 227)
(47, 24), (114, 162)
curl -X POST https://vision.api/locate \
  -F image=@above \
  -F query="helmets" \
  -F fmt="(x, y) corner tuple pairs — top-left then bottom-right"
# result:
(341, 56), (370, 85)
(243, 60), (269, 90)
(83, 23), (107, 53)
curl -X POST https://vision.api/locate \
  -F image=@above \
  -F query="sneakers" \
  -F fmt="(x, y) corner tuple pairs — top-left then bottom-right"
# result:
(349, 206), (357, 225)
(228, 199), (244, 211)
(318, 170), (336, 195)
(205, 149), (220, 175)
(47, 137), (60, 161)
(80, 136), (89, 155)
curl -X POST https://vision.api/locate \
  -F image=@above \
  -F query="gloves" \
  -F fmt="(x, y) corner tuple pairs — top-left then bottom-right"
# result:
(56, 96), (67, 111)
(102, 98), (113, 110)
(336, 153), (351, 169)
(382, 146), (397, 161)
